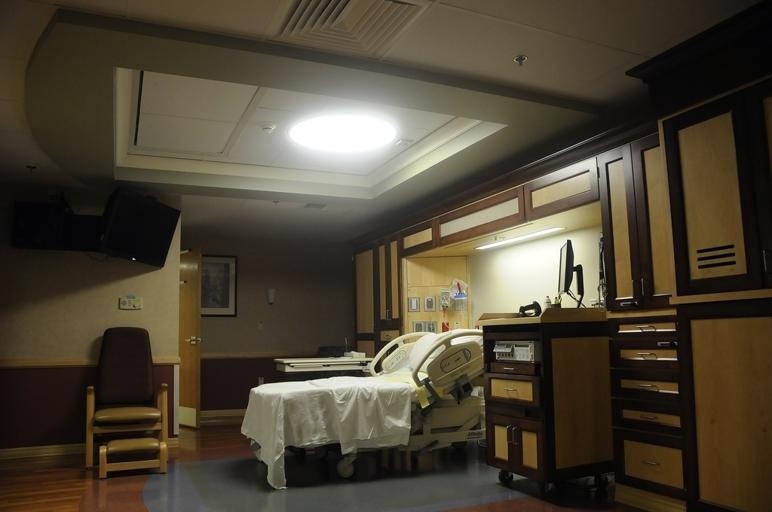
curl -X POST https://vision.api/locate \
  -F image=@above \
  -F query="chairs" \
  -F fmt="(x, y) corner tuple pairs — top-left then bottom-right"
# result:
(84, 327), (170, 469)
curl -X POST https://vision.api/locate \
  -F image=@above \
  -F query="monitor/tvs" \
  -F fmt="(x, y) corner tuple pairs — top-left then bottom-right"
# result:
(100, 186), (183, 269)
(556, 237), (584, 294)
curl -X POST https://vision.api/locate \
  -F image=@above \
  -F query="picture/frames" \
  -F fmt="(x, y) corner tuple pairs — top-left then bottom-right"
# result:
(201, 255), (238, 318)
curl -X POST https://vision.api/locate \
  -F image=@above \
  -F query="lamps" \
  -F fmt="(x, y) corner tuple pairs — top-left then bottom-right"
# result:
(289, 110), (397, 155)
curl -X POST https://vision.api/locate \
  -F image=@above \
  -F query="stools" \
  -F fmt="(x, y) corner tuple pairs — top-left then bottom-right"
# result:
(98, 437), (167, 479)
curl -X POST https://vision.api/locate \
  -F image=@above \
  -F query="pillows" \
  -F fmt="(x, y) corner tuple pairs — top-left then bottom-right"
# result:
(409, 334), (466, 372)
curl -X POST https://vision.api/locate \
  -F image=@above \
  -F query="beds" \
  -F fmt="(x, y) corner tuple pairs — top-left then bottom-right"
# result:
(246, 329), (486, 480)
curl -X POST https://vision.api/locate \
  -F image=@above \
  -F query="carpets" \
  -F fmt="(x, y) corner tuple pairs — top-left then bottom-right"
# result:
(143, 455), (545, 512)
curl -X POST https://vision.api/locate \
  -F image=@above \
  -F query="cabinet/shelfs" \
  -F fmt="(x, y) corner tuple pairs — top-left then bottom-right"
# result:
(476, 307), (624, 497)
(375, 235), (403, 325)
(610, 310), (692, 512)
(374, 325), (404, 374)
(597, 130), (673, 311)
(666, 63), (772, 297)
